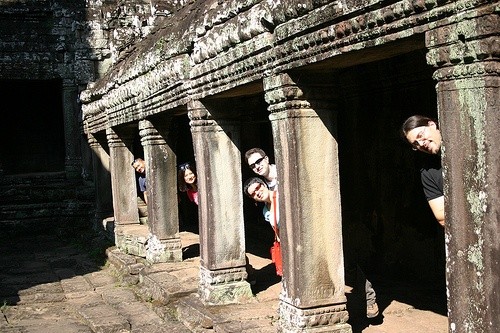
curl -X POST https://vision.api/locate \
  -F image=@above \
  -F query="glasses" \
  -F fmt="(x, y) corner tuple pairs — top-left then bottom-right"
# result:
(248, 155), (267, 169)
(249, 183), (262, 198)
(131, 160), (137, 166)
(178, 162), (190, 172)
(412, 126), (426, 151)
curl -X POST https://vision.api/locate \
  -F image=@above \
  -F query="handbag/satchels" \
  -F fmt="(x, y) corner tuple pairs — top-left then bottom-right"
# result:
(270, 239), (283, 276)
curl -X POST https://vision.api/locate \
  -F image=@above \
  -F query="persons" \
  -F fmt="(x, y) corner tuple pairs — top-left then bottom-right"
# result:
(178, 163), (198, 207)
(402, 116), (445, 227)
(243, 148), (281, 243)
(345, 253), (380, 319)
(131, 158), (148, 205)
